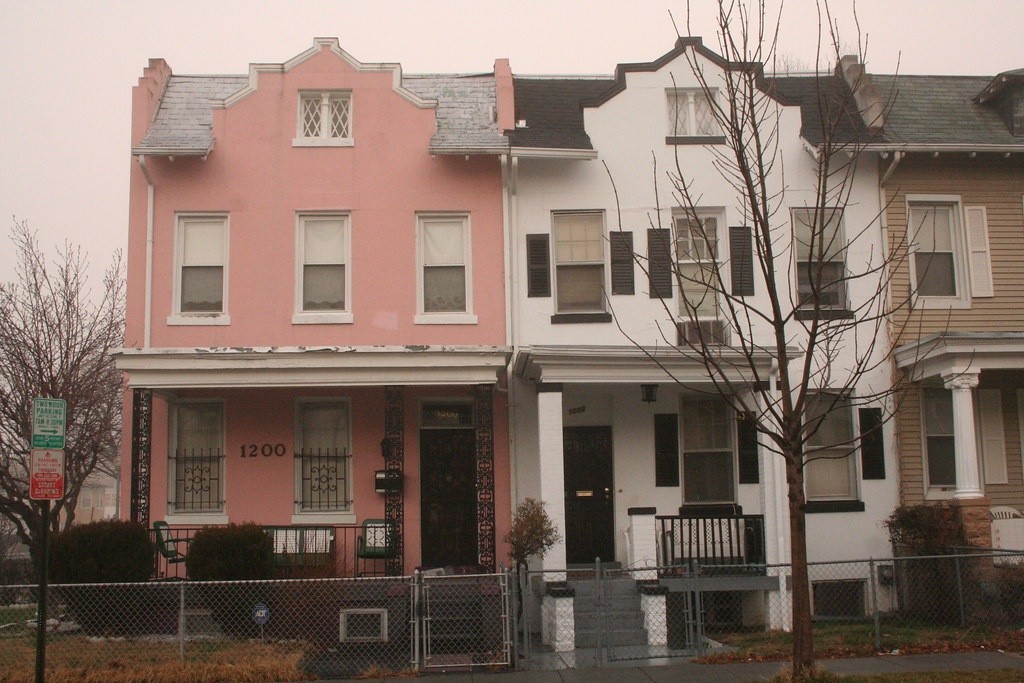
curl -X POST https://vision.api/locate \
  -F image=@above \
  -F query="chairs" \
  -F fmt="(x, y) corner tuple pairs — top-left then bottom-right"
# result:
(625, 524), (659, 569)
(356, 520), (398, 577)
(990, 507), (1022, 519)
(153, 521), (196, 579)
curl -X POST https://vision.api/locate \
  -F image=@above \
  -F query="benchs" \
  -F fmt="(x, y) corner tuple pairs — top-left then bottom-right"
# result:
(258, 526), (337, 578)
(663, 518), (758, 564)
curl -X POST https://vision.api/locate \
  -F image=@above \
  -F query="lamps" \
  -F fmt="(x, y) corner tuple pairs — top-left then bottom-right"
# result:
(380, 437), (393, 460)
(641, 383), (658, 405)
